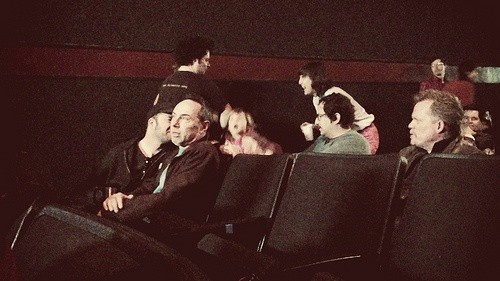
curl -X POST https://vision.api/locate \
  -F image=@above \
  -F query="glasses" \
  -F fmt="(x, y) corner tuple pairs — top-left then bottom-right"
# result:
(315, 113), (329, 121)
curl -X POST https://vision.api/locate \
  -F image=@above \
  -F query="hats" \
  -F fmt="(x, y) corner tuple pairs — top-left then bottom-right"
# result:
(145, 104), (175, 121)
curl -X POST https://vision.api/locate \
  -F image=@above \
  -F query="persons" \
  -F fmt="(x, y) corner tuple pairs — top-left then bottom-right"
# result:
(97, 36), (379, 223)
(393, 56), (500, 231)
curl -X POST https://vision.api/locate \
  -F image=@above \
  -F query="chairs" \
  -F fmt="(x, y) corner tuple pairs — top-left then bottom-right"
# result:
(10, 153), (500, 281)
